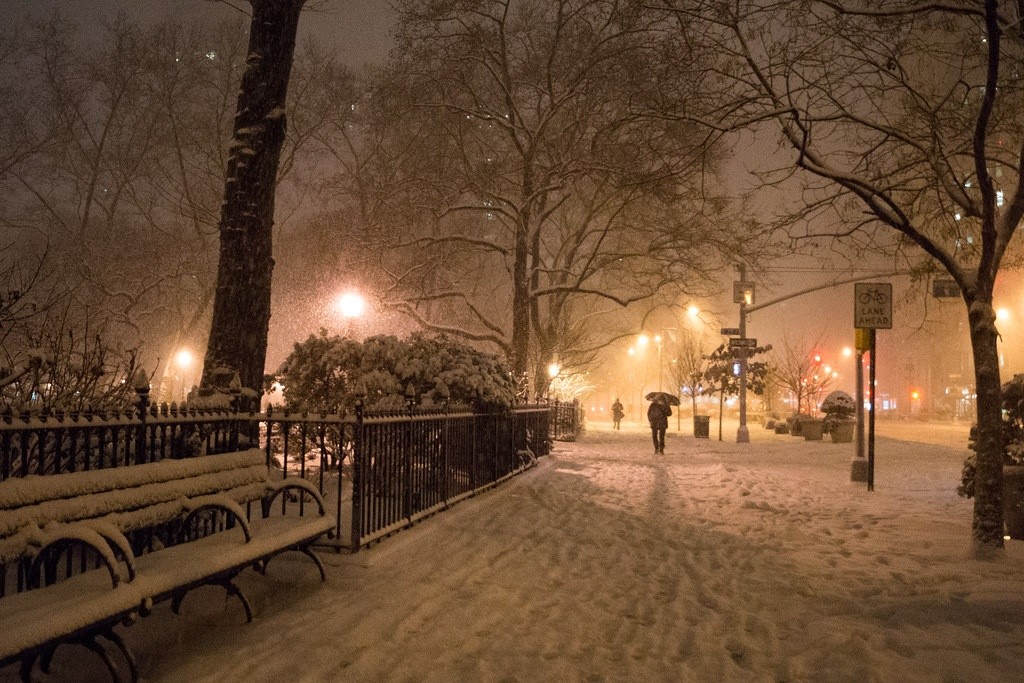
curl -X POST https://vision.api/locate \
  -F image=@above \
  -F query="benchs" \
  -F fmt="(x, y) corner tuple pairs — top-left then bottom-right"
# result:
(0, 448), (338, 683)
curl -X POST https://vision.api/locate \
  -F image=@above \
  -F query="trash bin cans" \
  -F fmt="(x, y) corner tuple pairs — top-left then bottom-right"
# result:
(693, 415), (711, 438)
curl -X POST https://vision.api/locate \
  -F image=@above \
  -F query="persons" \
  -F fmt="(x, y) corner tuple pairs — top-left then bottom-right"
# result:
(611, 398), (625, 430)
(647, 402), (672, 456)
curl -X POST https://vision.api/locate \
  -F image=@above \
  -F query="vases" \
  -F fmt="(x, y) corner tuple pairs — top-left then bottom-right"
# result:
(799, 418), (825, 440)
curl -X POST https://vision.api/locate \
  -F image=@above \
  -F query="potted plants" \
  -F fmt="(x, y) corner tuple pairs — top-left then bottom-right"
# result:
(955, 372), (1024, 540)
(821, 391), (857, 443)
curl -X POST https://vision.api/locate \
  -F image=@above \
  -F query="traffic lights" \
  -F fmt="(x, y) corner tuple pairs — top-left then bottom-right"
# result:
(814, 351), (822, 365)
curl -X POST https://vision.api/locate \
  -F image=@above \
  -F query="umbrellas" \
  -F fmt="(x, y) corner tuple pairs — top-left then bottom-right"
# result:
(645, 392), (682, 407)
